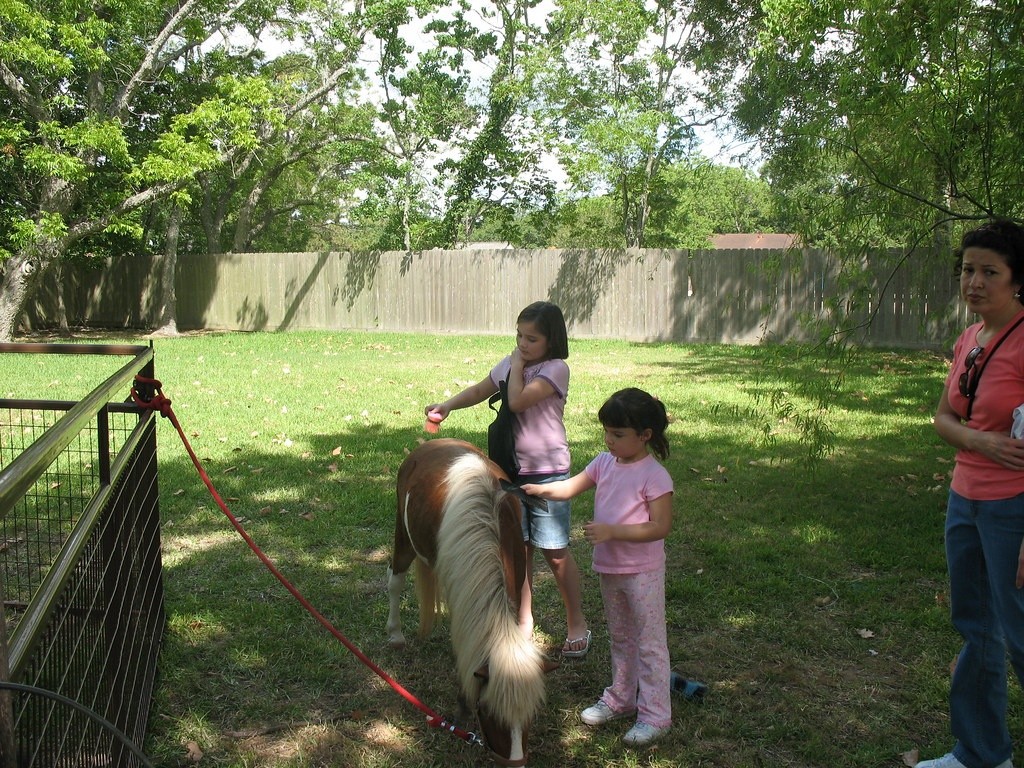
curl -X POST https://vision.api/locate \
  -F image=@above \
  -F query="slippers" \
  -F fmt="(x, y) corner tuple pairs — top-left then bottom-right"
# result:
(562, 630), (592, 657)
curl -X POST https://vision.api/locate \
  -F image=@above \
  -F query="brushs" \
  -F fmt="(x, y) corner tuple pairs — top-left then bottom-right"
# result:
(670, 670), (707, 702)
(424, 411), (443, 434)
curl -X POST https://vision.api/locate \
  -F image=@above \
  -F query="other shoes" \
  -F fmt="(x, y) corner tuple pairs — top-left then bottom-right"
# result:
(622, 721), (671, 746)
(914, 751), (1015, 768)
(581, 699), (635, 726)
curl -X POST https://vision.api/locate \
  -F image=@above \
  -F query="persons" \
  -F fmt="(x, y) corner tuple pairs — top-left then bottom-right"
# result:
(520, 387), (675, 746)
(911, 220), (1024, 768)
(425, 300), (592, 658)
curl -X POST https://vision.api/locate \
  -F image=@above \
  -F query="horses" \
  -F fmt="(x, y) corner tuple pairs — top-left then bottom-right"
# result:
(383, 439), (559, 768)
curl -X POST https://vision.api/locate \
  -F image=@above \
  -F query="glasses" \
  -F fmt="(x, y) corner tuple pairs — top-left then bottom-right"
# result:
(958, 346), (984, 398)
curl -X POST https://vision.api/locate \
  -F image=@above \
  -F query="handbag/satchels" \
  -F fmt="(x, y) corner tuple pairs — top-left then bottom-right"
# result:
(488, 367), (521, 482)
